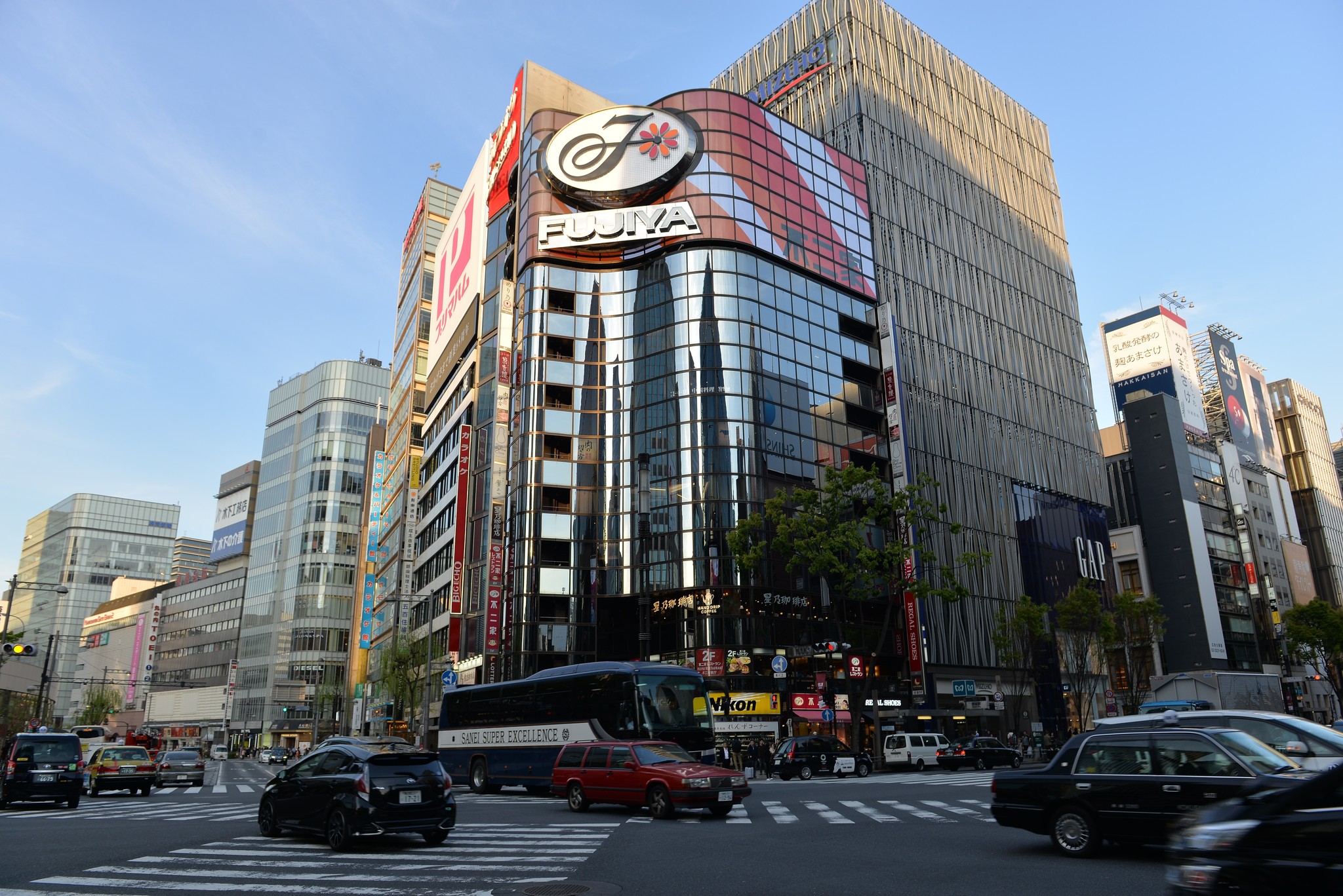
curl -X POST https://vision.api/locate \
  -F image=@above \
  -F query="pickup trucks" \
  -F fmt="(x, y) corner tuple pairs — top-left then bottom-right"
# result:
(69, 725), (118, 765)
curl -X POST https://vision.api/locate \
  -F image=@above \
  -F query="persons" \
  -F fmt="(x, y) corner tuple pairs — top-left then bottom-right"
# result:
(719, 742), (732, 769)
(1198, 743), (1226, 776)
(1007, 729), (1032, 758)
(240, 746), (311, 761)
(747, 739), (780, 780)
(1043, 727), (1079, 749)
(732, 735), (744, 771)
(987, 730), (998, 741)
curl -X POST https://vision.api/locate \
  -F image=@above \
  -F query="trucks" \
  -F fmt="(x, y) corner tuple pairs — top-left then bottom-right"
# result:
(122, 728), (164, 759)
(1139, 671), (1286, 715)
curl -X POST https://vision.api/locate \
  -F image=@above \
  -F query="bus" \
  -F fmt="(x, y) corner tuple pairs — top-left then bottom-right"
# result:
(438, 659), (731, 798)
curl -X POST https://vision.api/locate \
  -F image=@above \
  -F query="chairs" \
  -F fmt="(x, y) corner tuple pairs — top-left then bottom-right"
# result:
(132, 755), (141, 759)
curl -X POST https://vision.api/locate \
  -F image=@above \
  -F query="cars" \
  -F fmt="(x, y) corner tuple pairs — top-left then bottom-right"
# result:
(259, 750), (272, 764)
(990, 726), (1343, 896)
(935, 737), (1024, 772)
(82, 745), (157, 798)
(152, 746), (206, 788)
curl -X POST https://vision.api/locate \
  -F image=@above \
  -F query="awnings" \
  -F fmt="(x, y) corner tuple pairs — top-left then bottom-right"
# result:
(792, 711), (865, 723)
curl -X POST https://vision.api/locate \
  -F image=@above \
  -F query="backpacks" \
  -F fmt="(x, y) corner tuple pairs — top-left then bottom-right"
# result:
(1009, 735), (1014, 744)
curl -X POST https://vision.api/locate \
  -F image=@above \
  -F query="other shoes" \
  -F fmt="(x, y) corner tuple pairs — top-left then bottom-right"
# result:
(770, 778), (773, 780)
(755, 777), (759, 778)
(733, 769), (738, 771)
(773, 775), (776, 777)
(766, 778), (770, 781)
(764, 773), (766, 775)
(759, 773), (762, 775)
(740, 769), (744, 771)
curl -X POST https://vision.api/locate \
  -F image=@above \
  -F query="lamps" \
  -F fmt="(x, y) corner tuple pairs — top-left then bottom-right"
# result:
(1240, 459), (1270, 474)
(1202, 432), (1224, 448)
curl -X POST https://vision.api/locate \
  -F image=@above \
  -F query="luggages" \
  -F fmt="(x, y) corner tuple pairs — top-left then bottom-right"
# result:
(745, 757), (754, 779)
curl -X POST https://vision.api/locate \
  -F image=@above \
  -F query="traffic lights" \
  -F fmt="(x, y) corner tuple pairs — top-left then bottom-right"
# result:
(1304, 675), (1322, 681)
(283, 708), (294, 712)
(0, 643), (37, 657)
(230, 685), (256, 755)
(105, 709), (119, 715)
(811, 642), (838, 655)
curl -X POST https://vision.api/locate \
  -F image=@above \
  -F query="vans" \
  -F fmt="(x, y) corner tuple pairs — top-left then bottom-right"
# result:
(883, 733), (952, 772)
(268, 747), (288, 766)
(0, 732), (87, 809)
(209, 744), (229, 762)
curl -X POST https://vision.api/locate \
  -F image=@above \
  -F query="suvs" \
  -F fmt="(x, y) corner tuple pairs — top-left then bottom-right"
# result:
(256, 735), (457, 853)
(768, 736), (874, 781)
(1090, 708), (1343, 759)
(547, 738), (753, 819)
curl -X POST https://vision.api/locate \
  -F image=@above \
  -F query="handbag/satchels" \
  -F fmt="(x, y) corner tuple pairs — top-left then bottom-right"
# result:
(1027, 746), (1033, 756)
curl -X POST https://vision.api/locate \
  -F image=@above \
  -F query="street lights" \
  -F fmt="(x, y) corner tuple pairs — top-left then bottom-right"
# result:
(26, 634), (96, 726)
(135, 693), (157, 728)
(384, 589), (454, 747)
(85, 666), (132, 725)
(0, 574), (68, 658)
(317, 654), (358, 736)
